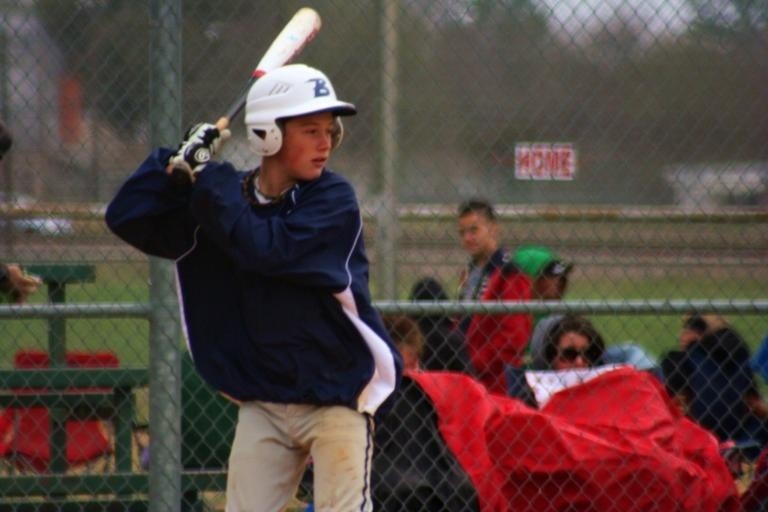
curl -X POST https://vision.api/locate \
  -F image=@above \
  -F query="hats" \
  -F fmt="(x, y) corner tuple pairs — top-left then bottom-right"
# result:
(530, 312), (565, 366)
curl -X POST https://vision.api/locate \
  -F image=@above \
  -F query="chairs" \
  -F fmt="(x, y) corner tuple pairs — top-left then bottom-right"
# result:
(1, 351), (128, 475)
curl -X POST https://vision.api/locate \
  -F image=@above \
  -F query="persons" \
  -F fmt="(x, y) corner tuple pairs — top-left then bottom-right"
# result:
(105, 65), (402, 512)
(1, 262), (44, 302)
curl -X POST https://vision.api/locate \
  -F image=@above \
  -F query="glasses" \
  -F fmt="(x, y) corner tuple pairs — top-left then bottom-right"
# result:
(562, 347), (598, 362)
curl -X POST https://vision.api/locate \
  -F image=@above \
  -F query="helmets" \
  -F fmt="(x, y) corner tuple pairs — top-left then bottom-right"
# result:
(243, 63), (357, 157)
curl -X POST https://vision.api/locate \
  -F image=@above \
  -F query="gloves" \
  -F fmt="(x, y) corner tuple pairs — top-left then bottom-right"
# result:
(169, 119), (231, 182)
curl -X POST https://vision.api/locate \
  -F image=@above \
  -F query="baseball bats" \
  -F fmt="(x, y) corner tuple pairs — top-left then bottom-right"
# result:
(165, 8), (323, 182)
